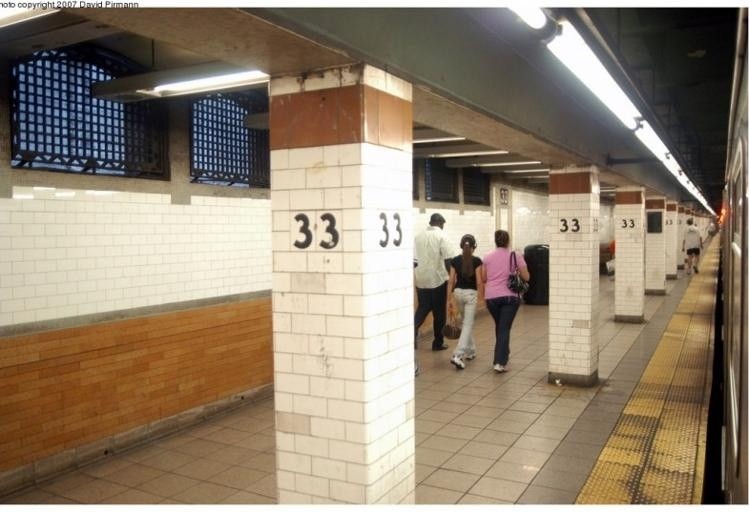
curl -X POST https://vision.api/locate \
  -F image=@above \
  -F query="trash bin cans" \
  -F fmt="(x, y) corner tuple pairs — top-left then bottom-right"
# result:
(524, 244), (548, 305)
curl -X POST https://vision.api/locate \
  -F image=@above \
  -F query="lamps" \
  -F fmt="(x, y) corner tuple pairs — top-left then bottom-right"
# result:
(471, 161), (539, 167)
(634, 120), (680, 177)
(0, 8), (270, 130)
(509, 7), (547, 30)
(430, 150), (509, 158)
(413, 136), (468, 143)
(542, 17), (642, 130)
(499, 168), (552, 180)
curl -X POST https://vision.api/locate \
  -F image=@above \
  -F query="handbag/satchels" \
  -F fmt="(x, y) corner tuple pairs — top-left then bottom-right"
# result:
(443, 311), (461, 339)
(508, 251), (530, 293)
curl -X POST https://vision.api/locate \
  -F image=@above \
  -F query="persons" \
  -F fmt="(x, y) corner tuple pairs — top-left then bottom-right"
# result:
(608, 237), (616, 283)
(412, 212), (452, 351)
(706, 219), (716, 241)
(481, 229), (532, 373)
(681, 219), (704, 276)
(445, 234), (486, 369)
(413, 258), (420, 377)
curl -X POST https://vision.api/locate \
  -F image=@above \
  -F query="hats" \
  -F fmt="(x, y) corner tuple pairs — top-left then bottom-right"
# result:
(429, 213), (445, 223)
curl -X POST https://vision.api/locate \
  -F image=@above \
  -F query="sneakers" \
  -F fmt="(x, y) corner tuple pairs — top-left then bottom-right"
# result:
(493, 363), (509, 372)
(687, 265), (699, 274)
(450, 345), (476, 369)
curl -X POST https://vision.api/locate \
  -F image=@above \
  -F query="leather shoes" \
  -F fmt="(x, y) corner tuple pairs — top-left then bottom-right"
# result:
(432, 344), (449, 350)
